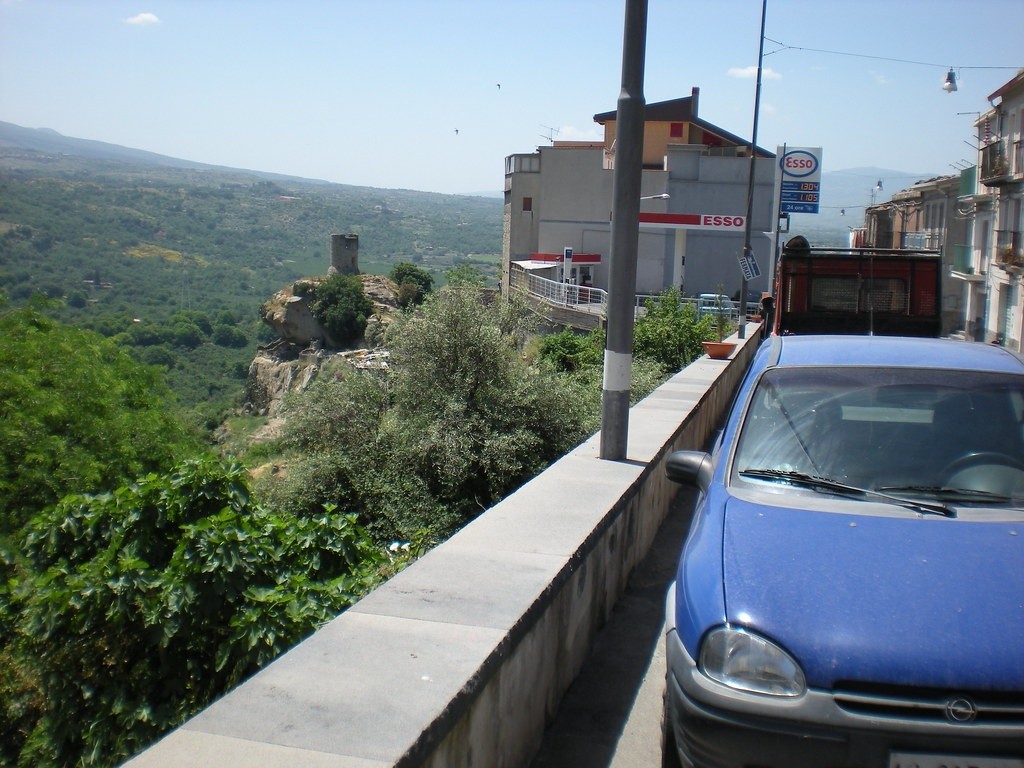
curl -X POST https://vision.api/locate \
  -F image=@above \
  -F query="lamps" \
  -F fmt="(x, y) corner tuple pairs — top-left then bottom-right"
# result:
(942, 66), (957, 94)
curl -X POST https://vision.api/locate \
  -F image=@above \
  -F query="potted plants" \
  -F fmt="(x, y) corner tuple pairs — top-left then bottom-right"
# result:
(993, 154), (1012, 176)
(996, 247), (1024, 274)
(702, 277), (738, 359)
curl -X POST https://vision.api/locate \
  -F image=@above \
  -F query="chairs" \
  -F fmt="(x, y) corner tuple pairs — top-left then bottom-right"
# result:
(933, 391), (1024, 485)
(766, 391), (859, 490)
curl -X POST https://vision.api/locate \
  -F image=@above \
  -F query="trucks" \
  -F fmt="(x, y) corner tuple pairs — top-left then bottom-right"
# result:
(759, 235), (943, 338)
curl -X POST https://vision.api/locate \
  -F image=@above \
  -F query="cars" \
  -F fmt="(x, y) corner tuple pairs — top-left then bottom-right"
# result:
(730, 289), (762, 320)
(665, 334), (1024, 768)
(699, 293), (738, 319)
